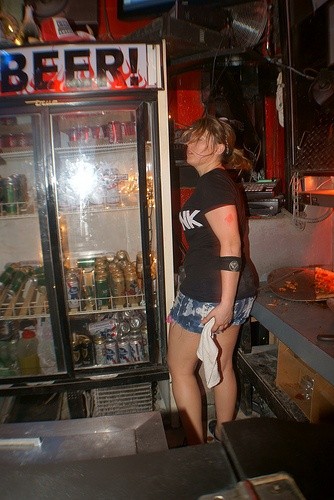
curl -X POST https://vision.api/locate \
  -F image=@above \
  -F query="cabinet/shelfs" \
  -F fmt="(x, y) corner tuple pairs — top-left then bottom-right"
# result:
(232, 281), (334, 423)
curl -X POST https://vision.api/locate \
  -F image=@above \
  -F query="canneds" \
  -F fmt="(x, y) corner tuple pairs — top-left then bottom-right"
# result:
(64, 249), (156, 309)
(68, 120), (136, 147)
(0, 174), (27, 216)
(87, 168), (121, 205)
(70, 307), (149, 369)
(0, 335), (20, 368)
(0, 131), (33, 149)
(0, 267), (46, 298)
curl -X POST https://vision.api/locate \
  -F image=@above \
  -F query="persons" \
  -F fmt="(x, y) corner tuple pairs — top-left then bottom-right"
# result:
(165, 114), (259, 447)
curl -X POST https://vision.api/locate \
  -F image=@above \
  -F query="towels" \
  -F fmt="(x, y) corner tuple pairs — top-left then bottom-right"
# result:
(196, 317), (222, 389)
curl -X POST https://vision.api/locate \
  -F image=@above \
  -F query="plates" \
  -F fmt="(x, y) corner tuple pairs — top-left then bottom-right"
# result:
(267, 266), (328, 301)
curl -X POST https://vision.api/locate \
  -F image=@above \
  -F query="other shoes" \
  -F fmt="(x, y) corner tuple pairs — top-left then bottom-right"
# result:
(207, 419), (221, 442)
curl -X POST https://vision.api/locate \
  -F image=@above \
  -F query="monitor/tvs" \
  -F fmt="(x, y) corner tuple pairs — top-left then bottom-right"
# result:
(117, 0), (177, 20)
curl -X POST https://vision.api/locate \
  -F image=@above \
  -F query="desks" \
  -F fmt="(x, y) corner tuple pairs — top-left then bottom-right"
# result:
(0, 411), (334, 500)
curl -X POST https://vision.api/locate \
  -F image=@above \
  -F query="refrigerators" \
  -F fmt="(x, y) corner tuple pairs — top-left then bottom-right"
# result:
(0, 38), (176, 396)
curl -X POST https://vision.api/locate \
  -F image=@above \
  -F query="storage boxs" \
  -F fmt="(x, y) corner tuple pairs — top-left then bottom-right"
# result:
(276, 339), (334, 424)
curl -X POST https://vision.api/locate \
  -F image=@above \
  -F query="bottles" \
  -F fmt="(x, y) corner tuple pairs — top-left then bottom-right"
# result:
(17, 328), (41, 375)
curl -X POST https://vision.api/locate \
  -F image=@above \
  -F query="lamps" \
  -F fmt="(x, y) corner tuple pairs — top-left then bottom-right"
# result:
(189, 0), (269, 55)
